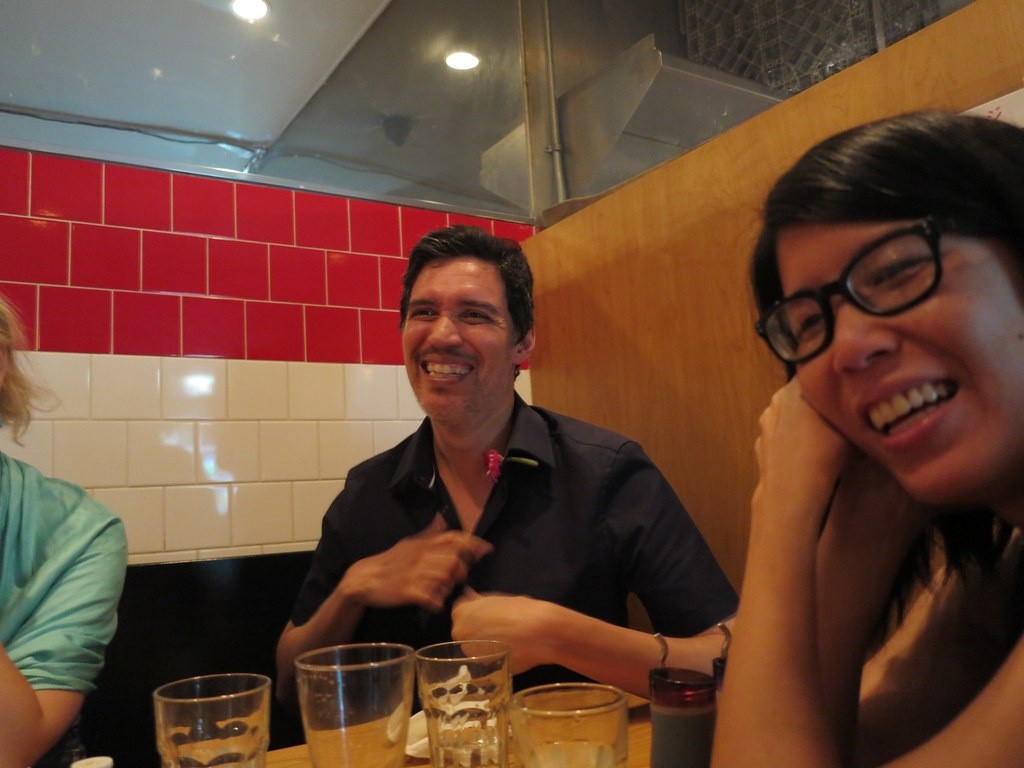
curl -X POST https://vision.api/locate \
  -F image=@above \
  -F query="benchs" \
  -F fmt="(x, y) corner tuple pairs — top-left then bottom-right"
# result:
(79, 550), (315, 768)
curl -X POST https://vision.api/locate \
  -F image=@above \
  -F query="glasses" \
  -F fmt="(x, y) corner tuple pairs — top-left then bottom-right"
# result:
(755, 215), (998, 363)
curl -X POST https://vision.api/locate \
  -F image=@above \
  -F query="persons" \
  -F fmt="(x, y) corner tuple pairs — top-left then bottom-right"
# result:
(273, 226), (736, 741)
(0, 292), (128, 768)
(711, 111), (1024, 768)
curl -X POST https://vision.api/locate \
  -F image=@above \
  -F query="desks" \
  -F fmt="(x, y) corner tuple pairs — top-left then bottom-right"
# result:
(265, 687), (652, 768)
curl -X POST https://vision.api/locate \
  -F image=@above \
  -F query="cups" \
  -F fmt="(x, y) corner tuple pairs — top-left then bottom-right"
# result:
(294, 644), (415, 768)
(507, 681), (629, 768)
(648, 667), (717, 768)
(152, 673), (271, 768)
(415, 639), (513, 768)
